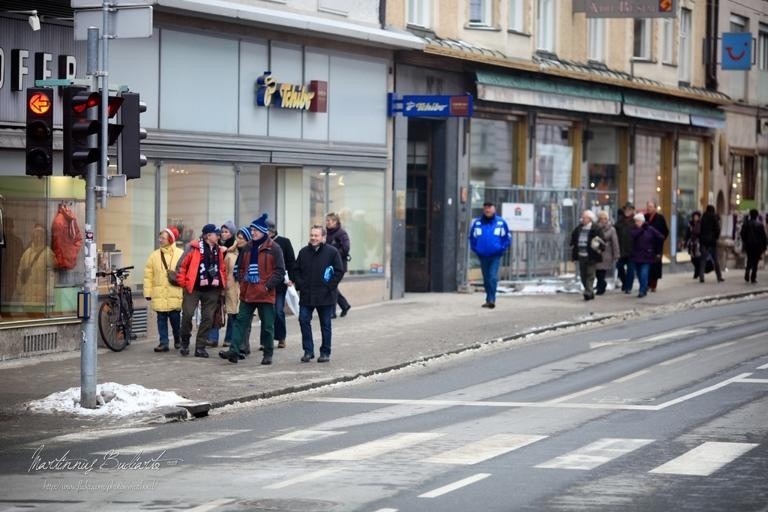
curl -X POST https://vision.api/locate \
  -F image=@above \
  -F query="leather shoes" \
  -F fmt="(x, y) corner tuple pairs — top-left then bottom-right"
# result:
(195, 347), (209, 358)
(180, 344), (192, 356)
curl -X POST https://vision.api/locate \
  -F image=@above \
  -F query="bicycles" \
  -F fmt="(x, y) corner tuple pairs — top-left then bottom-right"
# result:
(95, 265), (135, 354)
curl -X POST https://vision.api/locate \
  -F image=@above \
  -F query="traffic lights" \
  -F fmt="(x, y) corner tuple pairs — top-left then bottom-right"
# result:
(25, 91), (55, 171)
(123, 95), (146, 178)
(69, 92), (97, 180)
(109, 96), (123, 150)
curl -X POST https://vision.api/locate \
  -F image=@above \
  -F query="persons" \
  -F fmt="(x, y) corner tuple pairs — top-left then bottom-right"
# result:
(142, 213), (350, 363)
(15, 226), (56, 307)
(700, 205), (725, 282)
(683, 211), (701, 278)
(740, 209), (767, 283)
(469, 202), (511, 308)
(0, 217), (23, 319)
(569, 200), (670, 300)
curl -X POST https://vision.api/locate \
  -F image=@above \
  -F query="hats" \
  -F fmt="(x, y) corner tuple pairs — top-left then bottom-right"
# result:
(202, 223), (222, 234)
(250, 213), (269, 234)
(483, 200), (496, 206)
(622, 202), (636, 210)
(164, 227), (180, 244)
(222, 219), (237, 236)
(239, 226), (253, 241)
(269, 220), (276, 233)
(32, 222), (47, 232)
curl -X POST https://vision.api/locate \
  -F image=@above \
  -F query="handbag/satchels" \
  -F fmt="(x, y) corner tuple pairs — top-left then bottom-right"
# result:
(212, 296), (224, 329)
(20, 268), (32, 284)
(167, 269), (181, 286)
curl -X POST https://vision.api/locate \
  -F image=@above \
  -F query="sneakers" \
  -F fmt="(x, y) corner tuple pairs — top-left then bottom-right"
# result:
(261, 352), (272, 364)
(154, 343), (170, 352)
(317, 355), (330, 362)
(340, 305), (351, 317)
(277, 340), (286, 348)
(173, 339), (181, 348)
(481, 302), (495, 308)
(259, 346), (264, 350)
(223, 342), (231, 347)
(218, 350), (238, 363)
(299, 353), (315, 362)
(207, 341), (218, 347)
